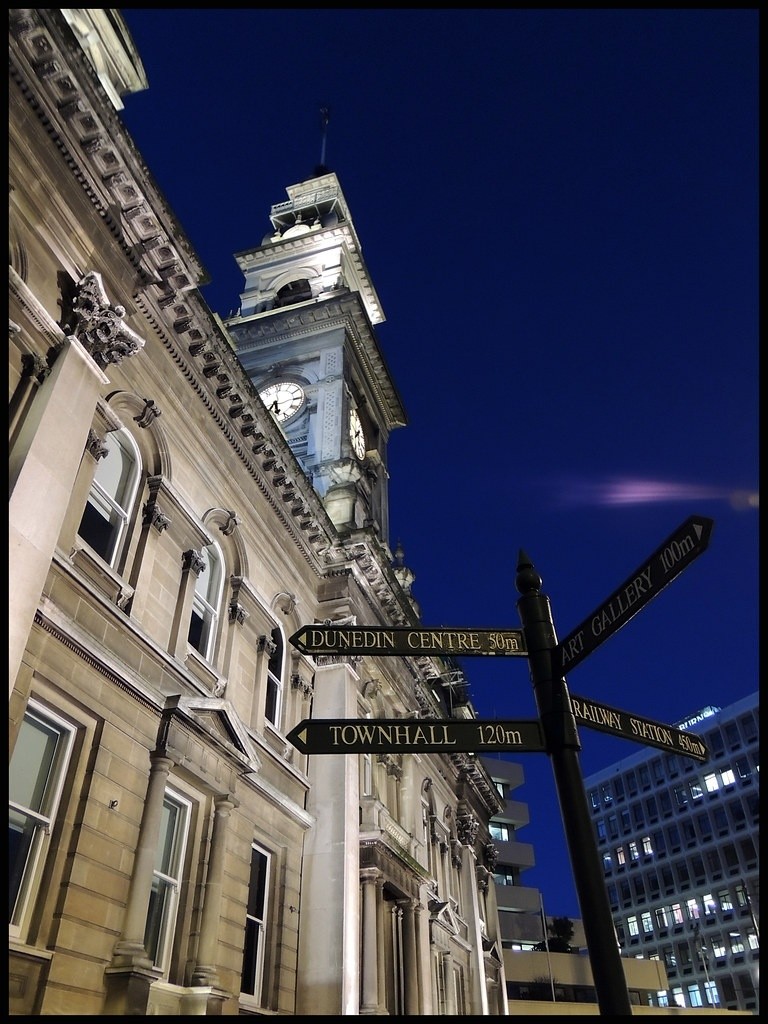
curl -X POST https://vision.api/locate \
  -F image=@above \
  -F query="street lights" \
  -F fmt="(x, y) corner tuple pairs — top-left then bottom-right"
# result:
(699, 947), (716, 1008)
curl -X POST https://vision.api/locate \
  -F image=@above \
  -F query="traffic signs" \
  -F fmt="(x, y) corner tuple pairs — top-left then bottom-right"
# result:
(571, 699), (711, 762)
(288, 624), (524, 657)
(544, 514), (712, 682)
(285, 719), (548, 755)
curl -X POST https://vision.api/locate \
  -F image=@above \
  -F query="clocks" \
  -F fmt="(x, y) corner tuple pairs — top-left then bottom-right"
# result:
(349, 409), (366, 460)
(258, 381), (305, 421)
